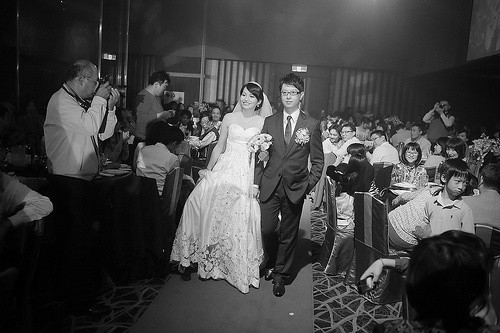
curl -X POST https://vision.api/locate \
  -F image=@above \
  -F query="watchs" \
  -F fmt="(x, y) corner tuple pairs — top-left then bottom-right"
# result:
(8, 219), (14, 231)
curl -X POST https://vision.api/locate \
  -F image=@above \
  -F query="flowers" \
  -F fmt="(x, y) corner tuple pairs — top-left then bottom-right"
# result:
(247, 133), (274, 164)
(294, 127), (311, 147)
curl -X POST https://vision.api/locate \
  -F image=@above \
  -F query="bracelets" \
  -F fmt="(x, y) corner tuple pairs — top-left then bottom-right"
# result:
(380, 258), (385, 267)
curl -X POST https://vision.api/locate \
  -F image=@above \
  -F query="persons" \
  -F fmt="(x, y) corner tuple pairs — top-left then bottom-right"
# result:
(44, 60), (121, 316)
(104, 71), (500, 288)
(372, 230), (500, 333)
(170, 81), (274, 293)
(254, 73), (324, 296)
(0, 171), (54, 273)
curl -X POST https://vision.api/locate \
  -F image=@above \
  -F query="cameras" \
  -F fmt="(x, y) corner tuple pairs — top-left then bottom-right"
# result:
(436, 104), (443, 109)
(358, 275), (373, 294)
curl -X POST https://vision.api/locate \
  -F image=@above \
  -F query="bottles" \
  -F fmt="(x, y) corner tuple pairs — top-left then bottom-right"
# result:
(398, 141), (405, 155)
(4, 145), (33, 166)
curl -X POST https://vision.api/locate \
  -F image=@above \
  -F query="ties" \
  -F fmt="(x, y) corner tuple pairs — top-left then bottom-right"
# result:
(285, 116), (292, 149)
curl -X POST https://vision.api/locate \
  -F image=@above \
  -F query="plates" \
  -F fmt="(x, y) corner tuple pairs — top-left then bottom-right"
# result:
(391, 189), (412, 195)
(120, 164), (128, 167)
(392, 182), (416, 189)
(424, 181), (439, 185)
(102, 168), (129, 174)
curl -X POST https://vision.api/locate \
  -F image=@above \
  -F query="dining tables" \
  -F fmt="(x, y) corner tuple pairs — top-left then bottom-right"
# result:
(94, 164), (134, 265)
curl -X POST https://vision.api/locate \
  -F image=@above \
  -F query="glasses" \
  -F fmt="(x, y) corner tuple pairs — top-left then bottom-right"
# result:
(406, 151), (418, 156)
(280, 89), (301, 96)
(340, 130), (353, 135)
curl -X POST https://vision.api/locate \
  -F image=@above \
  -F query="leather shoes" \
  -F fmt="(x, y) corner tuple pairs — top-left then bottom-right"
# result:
(265, 267), (275, 281)
(272, 275), (285, 297)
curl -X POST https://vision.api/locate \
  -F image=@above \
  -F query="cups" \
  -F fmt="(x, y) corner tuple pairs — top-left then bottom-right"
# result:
(97, 152), (111, 172)
(40, 153), (47, 166)
(394, 164), (428, 191)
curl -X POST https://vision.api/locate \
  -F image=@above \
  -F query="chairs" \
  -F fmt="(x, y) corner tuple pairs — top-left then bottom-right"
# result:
(0, 140), (218, 333)
(312, 159), (500, 333)
